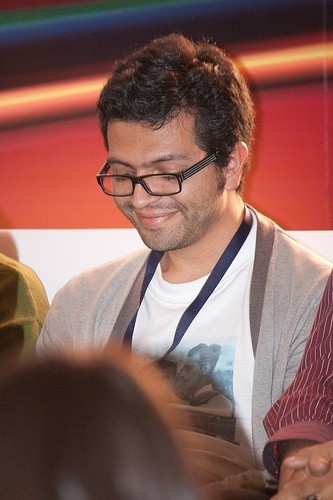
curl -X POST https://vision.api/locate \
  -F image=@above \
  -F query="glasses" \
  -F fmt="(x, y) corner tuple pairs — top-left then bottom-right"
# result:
(95, 152), (219, 197)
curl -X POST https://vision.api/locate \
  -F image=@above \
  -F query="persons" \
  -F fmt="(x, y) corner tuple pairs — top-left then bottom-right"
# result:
(33, 35), (332, 500)
(1, 358), (207, 500)
(259, 270), (333, 499)
(0, 253), (49, 372)
